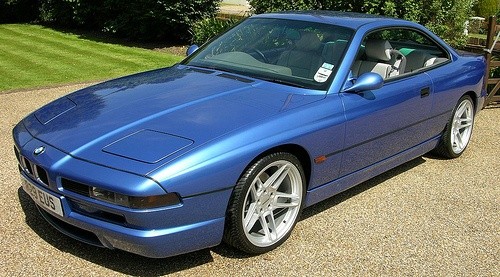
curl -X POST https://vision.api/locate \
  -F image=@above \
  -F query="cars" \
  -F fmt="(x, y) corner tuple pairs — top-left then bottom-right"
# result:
(12, 10), (488, 259)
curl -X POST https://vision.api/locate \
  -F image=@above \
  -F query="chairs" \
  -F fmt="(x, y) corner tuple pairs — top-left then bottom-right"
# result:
(279, 31), (446, 78)
